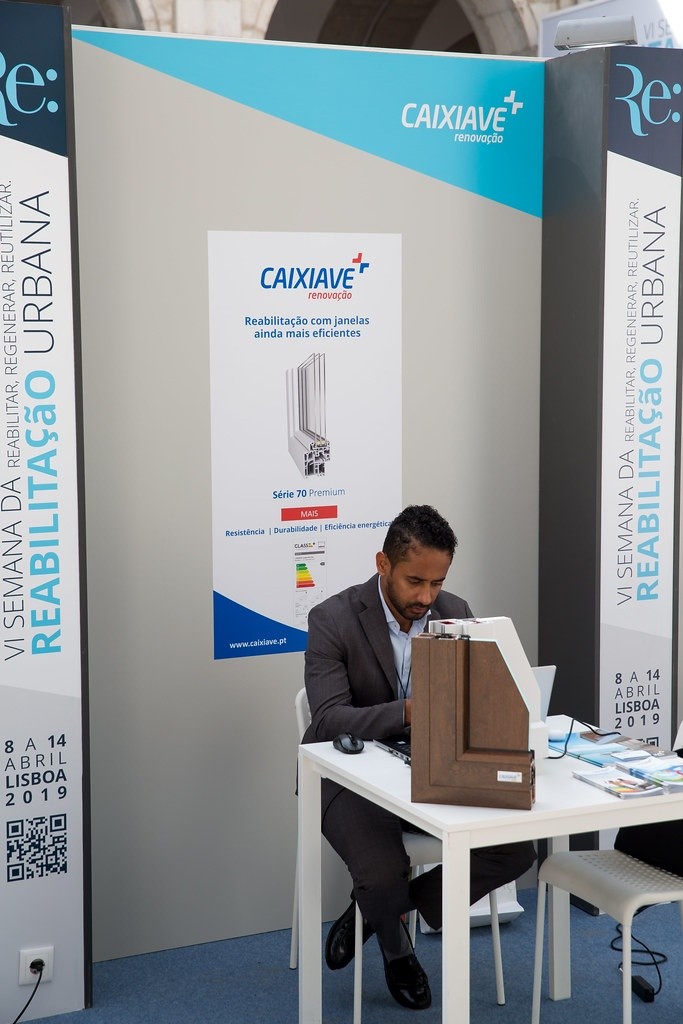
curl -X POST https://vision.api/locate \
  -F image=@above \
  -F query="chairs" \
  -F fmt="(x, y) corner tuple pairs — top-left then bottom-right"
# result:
(530, 716), (681, 1023)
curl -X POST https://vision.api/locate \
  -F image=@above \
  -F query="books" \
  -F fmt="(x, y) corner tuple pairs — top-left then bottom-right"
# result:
(548, 729), (678, 769)
(573, 766), (663, 798)
(616, 754), (683, 793)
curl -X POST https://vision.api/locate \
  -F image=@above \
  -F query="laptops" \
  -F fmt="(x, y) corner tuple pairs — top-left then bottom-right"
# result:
(373, 665), (557, 766)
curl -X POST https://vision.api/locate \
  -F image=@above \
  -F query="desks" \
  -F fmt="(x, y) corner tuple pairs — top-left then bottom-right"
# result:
(291, 710), (683, 1023)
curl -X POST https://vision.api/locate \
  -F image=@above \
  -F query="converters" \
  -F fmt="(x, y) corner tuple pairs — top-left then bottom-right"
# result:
(632, 975), (654, 1003)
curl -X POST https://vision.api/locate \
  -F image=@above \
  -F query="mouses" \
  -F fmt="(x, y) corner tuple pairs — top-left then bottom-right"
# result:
(333, 731), (364, 754)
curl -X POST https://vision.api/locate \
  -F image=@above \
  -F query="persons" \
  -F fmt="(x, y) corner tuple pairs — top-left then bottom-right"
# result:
(301, 504), (537, 1009)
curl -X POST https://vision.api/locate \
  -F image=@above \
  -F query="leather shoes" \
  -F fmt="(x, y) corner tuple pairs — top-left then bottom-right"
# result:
(379, 920), (431, 1011)
(324, 901), (372, 970)
